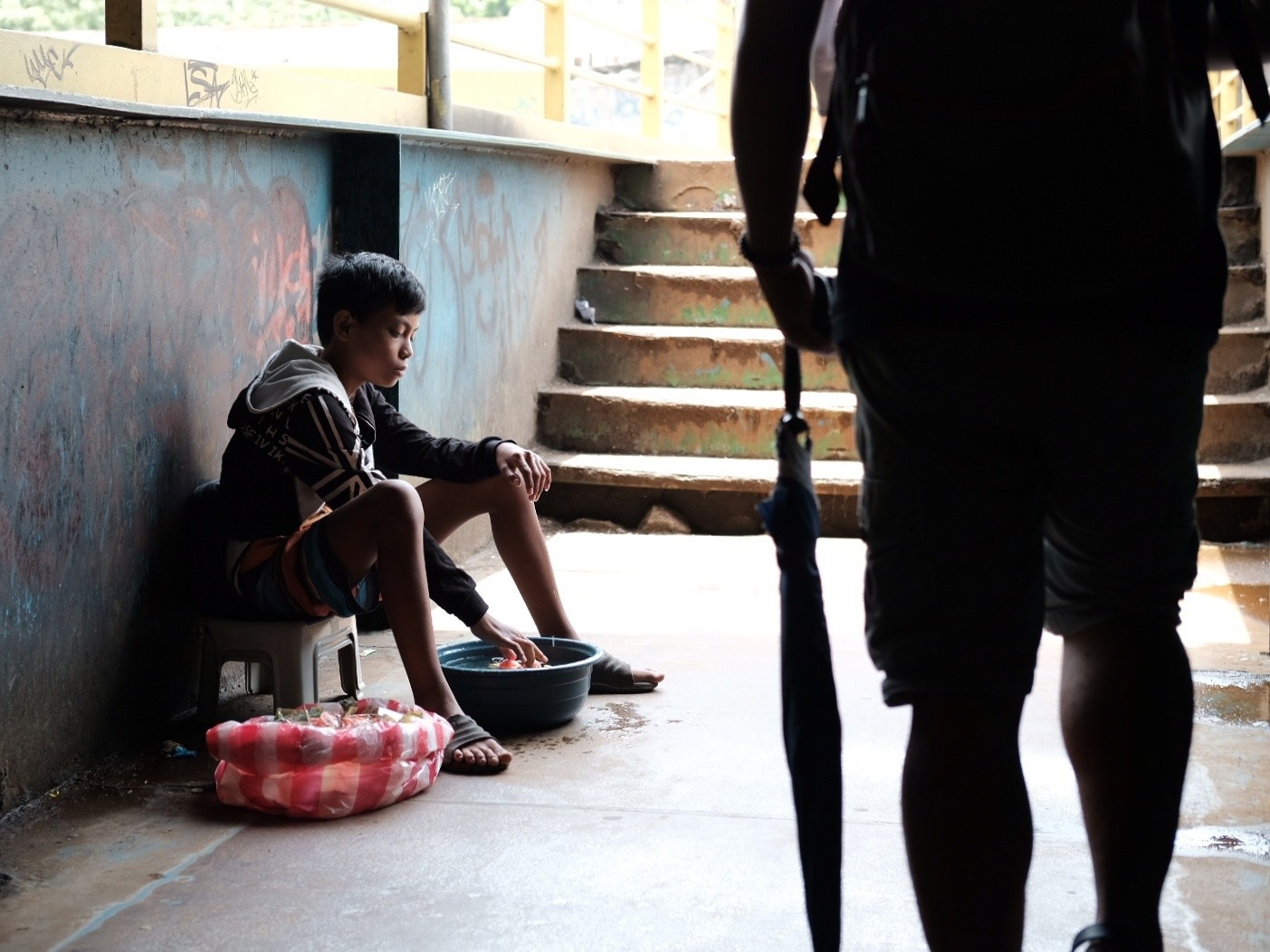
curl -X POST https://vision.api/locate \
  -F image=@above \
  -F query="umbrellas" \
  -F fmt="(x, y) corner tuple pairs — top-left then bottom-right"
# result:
(755, 274), (845, 951)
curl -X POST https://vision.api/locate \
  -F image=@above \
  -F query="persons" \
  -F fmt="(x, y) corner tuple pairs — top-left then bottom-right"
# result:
(222, 253), (665, 774)
(732, 0), (1270, 951)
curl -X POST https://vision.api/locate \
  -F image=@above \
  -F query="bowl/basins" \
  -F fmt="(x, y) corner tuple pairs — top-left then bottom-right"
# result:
(437, 636), (603, 729)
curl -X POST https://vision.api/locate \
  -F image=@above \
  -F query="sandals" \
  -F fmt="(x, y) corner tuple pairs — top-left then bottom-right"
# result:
(588, 650), (658, 694)
(443, 714), (508, 775)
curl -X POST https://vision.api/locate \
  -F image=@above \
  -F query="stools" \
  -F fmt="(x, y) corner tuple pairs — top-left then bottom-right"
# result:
(192, 615), (364, 730)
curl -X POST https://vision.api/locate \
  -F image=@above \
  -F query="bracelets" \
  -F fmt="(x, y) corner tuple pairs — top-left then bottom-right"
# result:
(741, 230), (802, 269)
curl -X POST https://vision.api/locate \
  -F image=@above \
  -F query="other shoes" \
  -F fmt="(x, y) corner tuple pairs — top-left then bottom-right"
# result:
(1071, 925), (1163, 952)
